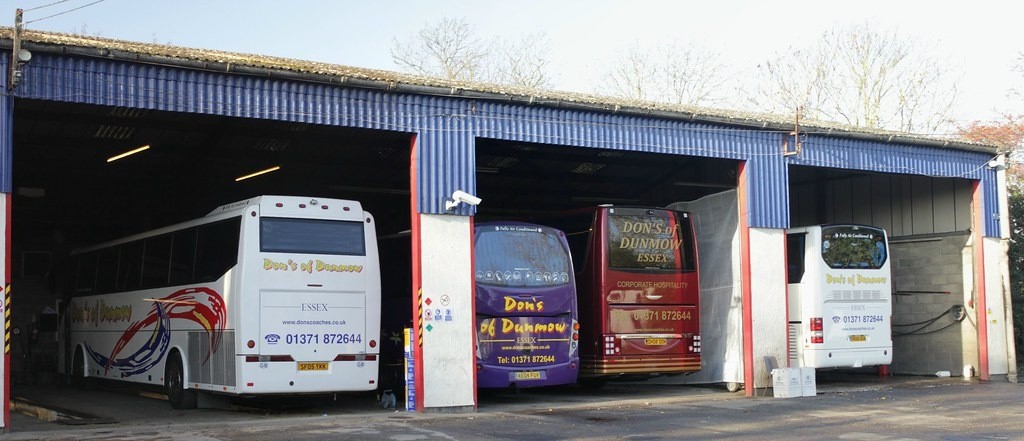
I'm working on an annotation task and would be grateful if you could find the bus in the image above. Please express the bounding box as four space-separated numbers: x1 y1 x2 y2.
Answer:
376 219 581 397
554 201 702 390
788 223 893 371
55 194 382 411
